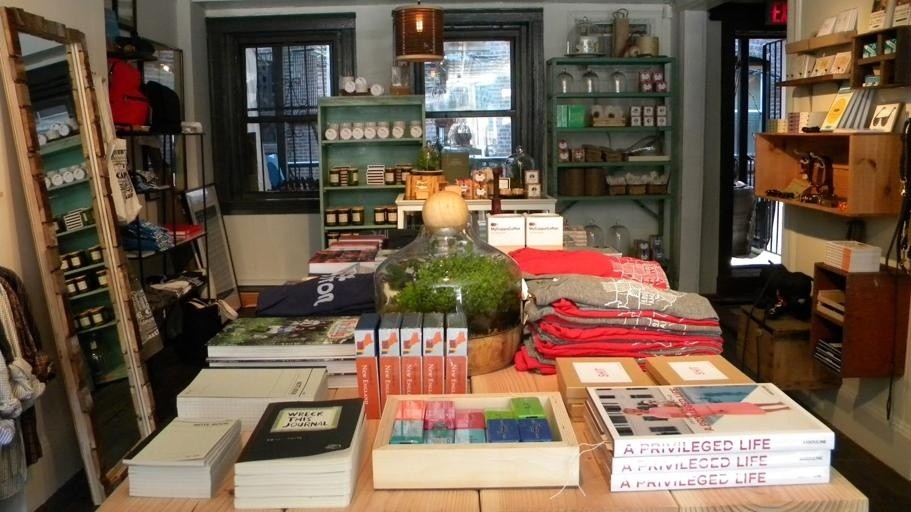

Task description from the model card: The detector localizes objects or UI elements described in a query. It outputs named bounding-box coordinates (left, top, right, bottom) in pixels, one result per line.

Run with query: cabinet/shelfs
left=116, top=133, right=208, bottom=369
left=546, top=57, right=676, bottom=282
left=809, top=262, right=910, bottom=377
left=16, top=47, right=129, bottom=387
left=317, top=95, right=425, bottom=252
left=753, top=1, right=910, bottom=219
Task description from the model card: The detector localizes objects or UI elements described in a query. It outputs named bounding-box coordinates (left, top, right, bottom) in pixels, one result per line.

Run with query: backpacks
left=106, top=55, right=149, bottom=128
left=145, top=80, right=183, bottom=136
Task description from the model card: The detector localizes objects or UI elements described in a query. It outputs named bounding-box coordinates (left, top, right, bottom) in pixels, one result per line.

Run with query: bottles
left=60, top=246, right=103, bottom=270
left=346, top=168, right=359, bottom=186
left=400, top=167, right=411, bottom=185
left=374, top=204, right=397, bottom=225
left=383, top=168, right=397, bottom=186
left=328, top=168, right=340, bottom=186
left=324, top=207, right=366, bottom=227
left=63, top=268, right=110, bottom=297
left=72, top=306, right=109, bottom=332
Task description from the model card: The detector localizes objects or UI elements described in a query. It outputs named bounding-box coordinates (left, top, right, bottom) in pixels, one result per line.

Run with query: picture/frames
left=183, top=184, right=242, bottom=326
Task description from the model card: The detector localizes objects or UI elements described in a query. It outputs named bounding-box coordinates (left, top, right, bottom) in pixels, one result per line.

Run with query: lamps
left=392, top=1, right=445, bottom=62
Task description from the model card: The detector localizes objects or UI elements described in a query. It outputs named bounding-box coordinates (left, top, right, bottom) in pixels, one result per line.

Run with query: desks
left=96, top=382, right=479, bottom=512
left=468, top=363, right=870, bottom=512
left=393, top=192, right=557, bottom=230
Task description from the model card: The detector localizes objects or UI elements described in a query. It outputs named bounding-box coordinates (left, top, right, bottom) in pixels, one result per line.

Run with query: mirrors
left=0, top=6, right=155, bottom=506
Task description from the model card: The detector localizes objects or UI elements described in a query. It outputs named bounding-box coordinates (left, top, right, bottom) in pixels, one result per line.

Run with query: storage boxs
left=736, top=305, right=826, bottom=395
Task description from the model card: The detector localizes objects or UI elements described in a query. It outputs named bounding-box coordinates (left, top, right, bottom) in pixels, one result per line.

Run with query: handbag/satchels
left=105, top=136, right=145, bottom=226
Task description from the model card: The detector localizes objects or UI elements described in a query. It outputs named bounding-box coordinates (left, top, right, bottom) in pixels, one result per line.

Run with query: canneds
left=401, top=166, right=412, bottom=183
left=352, top=208, right=364, bottom=224
left=387, top=207, right=397, bottom=224
left=328, top=234, right=338, bottom=245
left=71, top=304, right=107, bottom=331
left=330, top=168, right=341, bottom=186
left=338, top=208, right=348, bottom=224
left=348, top=167, right=360, bottom=184
left=57, top=245, right=112, bottom=293
left=384, top=168, right=395, bottom=184
left=326, top=209, right=335, bottom=225
left=373, top=208, right=386, bottom=225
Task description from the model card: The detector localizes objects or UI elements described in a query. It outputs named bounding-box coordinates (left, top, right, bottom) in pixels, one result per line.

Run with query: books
left=120, top=312, right=843, bottom=509
left=821, top=87, right=875, bottom=132
left=309, top=233, right=386, bottom=278
left=824, top=242, right=882, bottom=271
